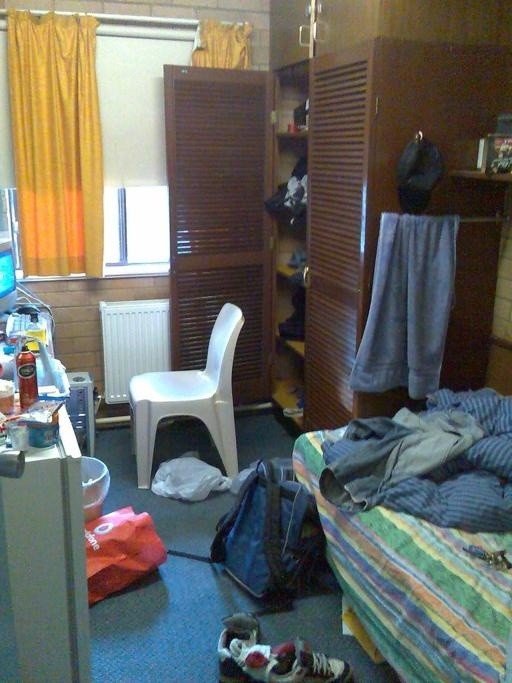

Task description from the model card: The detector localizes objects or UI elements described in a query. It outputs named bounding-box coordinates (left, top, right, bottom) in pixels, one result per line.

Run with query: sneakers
left=217, top=612, right=353, bottom=683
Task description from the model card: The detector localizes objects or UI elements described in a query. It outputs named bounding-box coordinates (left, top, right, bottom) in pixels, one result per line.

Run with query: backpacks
left=210, top=458, right=324, bottom=610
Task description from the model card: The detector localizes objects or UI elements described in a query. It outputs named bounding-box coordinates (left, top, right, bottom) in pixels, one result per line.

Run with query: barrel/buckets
left=80, top=455, right=109, bottom=523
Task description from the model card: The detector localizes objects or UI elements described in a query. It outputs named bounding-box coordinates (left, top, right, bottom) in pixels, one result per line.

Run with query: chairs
left=128, top=302, right=245, bottom=490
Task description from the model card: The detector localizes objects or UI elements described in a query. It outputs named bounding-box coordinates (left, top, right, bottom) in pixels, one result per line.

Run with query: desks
left=0, top=384, right=91, bottom=683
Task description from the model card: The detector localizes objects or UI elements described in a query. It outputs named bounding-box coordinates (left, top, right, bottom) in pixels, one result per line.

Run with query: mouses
left=15, top=305, right=40, bottom=313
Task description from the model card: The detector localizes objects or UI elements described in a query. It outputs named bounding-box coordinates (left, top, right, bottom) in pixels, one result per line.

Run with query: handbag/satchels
left=84, top=505, right=167, bottom=608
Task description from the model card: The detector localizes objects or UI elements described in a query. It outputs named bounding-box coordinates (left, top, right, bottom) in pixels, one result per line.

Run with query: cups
left=11, top=424, right=29, bottom=451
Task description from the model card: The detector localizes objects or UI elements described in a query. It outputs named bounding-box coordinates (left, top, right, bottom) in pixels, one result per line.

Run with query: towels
left=347, top=212, right=460, bottom=401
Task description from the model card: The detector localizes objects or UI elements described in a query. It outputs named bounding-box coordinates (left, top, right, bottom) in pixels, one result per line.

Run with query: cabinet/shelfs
left=163, top=2, right=512, bottom=439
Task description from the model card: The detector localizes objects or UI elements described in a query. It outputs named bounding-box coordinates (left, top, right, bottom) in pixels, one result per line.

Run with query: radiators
left=98, top=292, right=251, bottom=406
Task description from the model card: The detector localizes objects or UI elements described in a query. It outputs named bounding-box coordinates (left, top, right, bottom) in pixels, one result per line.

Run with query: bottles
left=0, top=313, right=46, bottom=414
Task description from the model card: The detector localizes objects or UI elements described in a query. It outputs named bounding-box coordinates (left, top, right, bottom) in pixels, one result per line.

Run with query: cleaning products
left=15, top=304, right=47, bottom=352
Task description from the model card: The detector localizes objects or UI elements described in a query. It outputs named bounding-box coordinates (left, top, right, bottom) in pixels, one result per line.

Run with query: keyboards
left=6, top=312, right=48, bottom=350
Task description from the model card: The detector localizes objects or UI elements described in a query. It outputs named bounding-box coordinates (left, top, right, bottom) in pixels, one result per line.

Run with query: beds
left=292, top=388, right=512, bottom=682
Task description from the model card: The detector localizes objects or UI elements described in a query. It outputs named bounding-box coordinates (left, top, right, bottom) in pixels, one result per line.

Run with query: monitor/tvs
left=0, top=238, right=18, bottom=315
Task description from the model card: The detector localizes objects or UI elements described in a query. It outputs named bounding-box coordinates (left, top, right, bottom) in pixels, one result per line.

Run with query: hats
left=398, top=134, right=443, bottom=214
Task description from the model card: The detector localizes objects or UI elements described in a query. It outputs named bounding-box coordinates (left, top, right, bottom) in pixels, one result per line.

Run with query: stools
left=66, top=367, right=95, bottom=457
